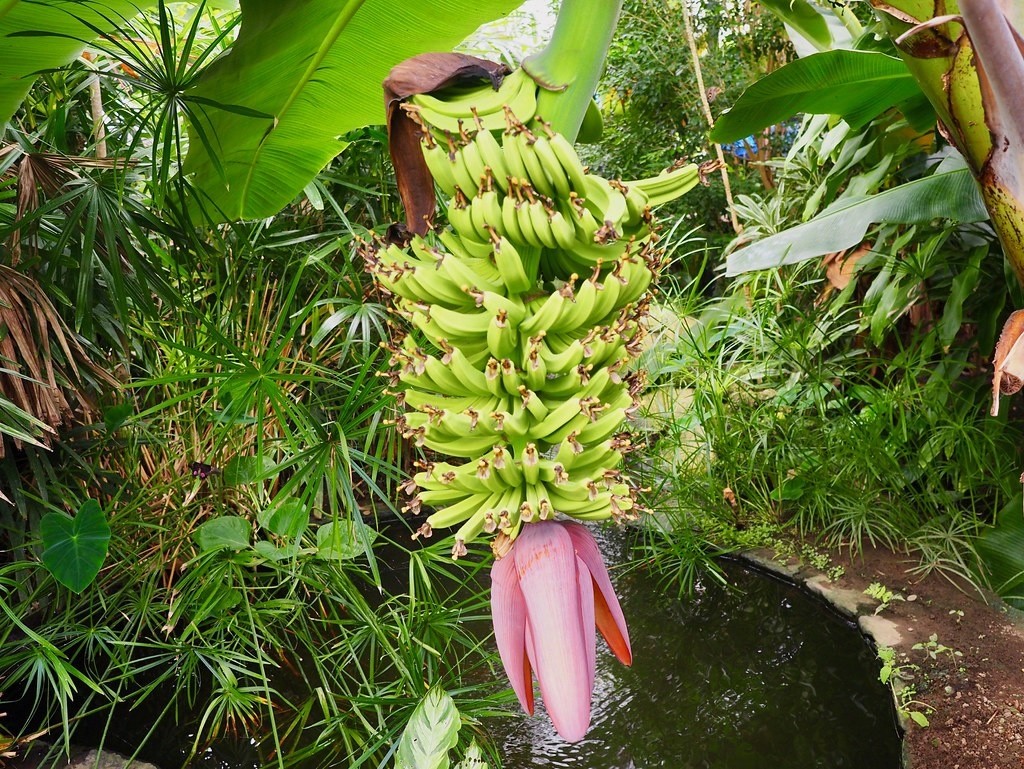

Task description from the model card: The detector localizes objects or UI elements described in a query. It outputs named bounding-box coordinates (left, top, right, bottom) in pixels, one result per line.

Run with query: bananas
left=355, top=65, right=722, bottom=564
